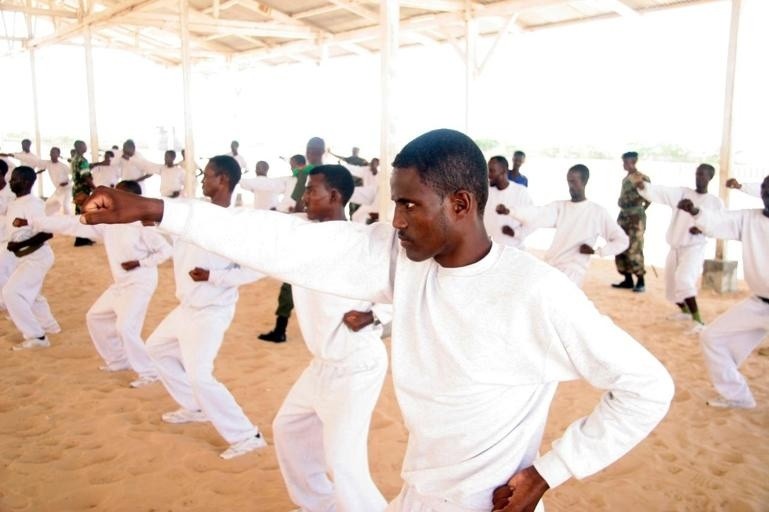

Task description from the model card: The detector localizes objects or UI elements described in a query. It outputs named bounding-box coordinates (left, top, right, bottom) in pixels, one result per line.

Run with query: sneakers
left=632, top=283, right=646, bottom=292
left=128, top=374, right=159, bottom=388
left=161, top=408, right=208, bottom=423
left=45, top=324, right=61, bottom=334
left=705, top=398, right=756, bottom=410
left=98, top=366, right=128, bottom=373
left=218, top=432, right=268, bottom=460
left=684, top=320, right=704, bottom=336
left=664, top=313, right=692, bottom=322
left=611, top=281, right=634, bottom=289
left=12, top=336, right=50, bottom=351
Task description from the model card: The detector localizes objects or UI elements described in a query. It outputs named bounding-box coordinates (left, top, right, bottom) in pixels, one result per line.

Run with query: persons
left=114, top=139, right=153, bottom=182
left=223, top=140, right=246, bottom=171
left=327, top=146, right=368, bottom=222
left=337, top=157, right=380, bottom=222
left=88, top=151, right=114, bottom=190
left=241, top=161, right=278, bottom=212
left=71, top=141, right=96, bottom=246
left=0, top=168, right=62, bottom=352
left=289, top=154, right=305, bottom=174
left=508, top=150, right=528, bottom=188
left=677, top=175, right=769, bottom=410
left=12, top=181, right=174, bottom=389
left=0, top=159, right=15, bottom=305
left=142, top=156, right=268, bottom=460
left=25, top=147, right=71, bottom=217
left=190, top=165, right=390, bottom=512
left=131, top=150, right=183, bottom=198
left=483, top=156, right=540, bottom=249
left=611, top=152, right=653, bottom=293
left=257, top=136, right=324, bottom=342
left=725, top=178, right=761, bottom=198
left=80, top=129, right=674, bottom=512
left=496, top=163, right=629, bottom=288
left=175, top=148, right=202, bottom=197
left=0, top=140, right=37, bottom=172
left=629, top=164, right=724, bottom=324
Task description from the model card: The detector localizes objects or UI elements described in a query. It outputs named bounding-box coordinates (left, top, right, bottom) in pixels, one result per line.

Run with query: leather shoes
left=258, top=331, right=286, bottom=344
